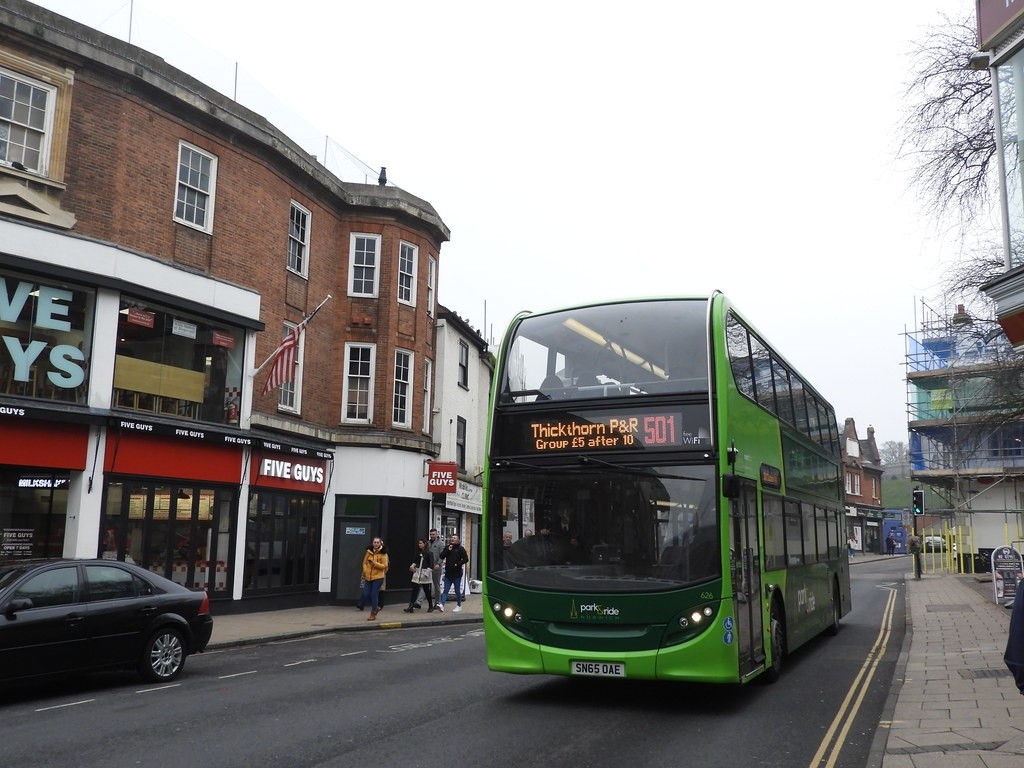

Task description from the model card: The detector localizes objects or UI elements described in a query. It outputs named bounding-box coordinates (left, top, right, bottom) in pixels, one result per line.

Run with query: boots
left=404, top=602, right=413, bottom=613
left=427, top=603, right=433, bottom=612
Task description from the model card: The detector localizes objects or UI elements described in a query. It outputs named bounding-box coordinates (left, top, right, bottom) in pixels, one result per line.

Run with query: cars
left=921, top=536, right=946, bottom=553
left=0, top=557, right=214, bottom=694
left=952, top=543, right=957, bottom=561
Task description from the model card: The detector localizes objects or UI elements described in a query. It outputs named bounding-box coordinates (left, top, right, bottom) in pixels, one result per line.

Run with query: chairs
left=535, top=362, right=701, bottom=401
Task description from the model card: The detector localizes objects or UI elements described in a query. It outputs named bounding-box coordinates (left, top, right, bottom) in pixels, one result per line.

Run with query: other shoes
left=413, top=603, right=421, bottom=609
left=368, top=611, right=376, bottom=621
left=375, top=606, right=381, bottom=615
left=433, top=602, right=440, bottom=609
left=378, top=603, right=384, bottom=609
left=356, top=603, right=365, bottom=611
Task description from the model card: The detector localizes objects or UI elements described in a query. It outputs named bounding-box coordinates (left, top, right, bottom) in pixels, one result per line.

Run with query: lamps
left=177, top=489, right=190, bottom=500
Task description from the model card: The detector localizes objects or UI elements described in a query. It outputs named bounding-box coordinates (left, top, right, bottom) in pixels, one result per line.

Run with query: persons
left=524, top=529, right=532, bottom=537
left=355, top=536, right=389, bottom=621
left=846, top=536, right=857, bottom=558
left=403, top=528, right=469, bottom=613
left=885, top=534, right=896, bottom=556
left=502, top=531, right=513, bottom=546
left=541, top=500, right=584, bottom=566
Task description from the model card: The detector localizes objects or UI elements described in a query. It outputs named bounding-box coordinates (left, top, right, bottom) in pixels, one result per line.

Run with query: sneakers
left=453, top=605, right=461, bottom=612
left=436, top=604, right=445, bottom=612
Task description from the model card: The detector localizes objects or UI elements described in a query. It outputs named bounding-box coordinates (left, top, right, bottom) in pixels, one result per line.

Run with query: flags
left=260, top=307, right=318, bottom=400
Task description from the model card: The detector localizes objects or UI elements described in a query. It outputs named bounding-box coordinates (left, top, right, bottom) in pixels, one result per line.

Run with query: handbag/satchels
left=893, top=540, right=897, bottom=546
left=848, top=539, right=851, bottom=549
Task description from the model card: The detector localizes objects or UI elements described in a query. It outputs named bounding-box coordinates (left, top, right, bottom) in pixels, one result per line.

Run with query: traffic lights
left=914, top=491, right=924, bottom=516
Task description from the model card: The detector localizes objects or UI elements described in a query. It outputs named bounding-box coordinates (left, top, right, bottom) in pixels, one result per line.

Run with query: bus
left=481, top=288, right=851, bottom=691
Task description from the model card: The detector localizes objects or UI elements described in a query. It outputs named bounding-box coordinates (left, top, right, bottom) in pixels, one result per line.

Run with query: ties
left=562, top=526, right=568, bottom=534
